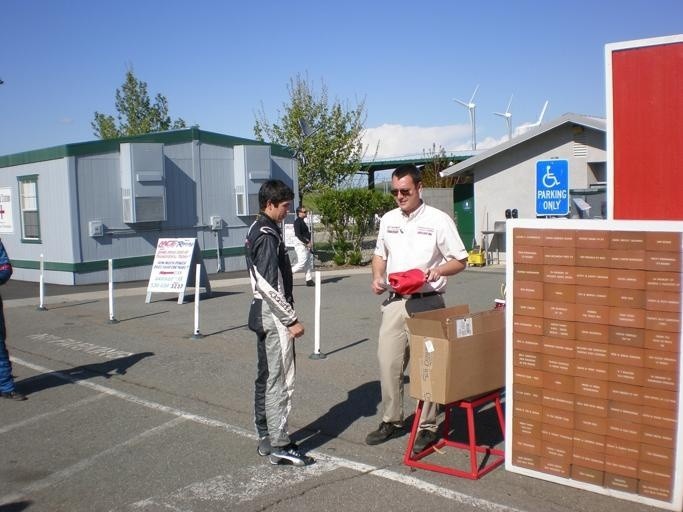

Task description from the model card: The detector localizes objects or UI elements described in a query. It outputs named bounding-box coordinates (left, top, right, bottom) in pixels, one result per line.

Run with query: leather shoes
left=389, top=290, right=442, bottom=298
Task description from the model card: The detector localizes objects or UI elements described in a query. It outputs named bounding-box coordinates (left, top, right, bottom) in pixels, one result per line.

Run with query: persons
left=0, top=239, right=23, bottom=400
left=366, top=163, right=467, bottom=453
left=244, top=181, right=315, bottom=465
left=289, top=206, right=315, bottom=287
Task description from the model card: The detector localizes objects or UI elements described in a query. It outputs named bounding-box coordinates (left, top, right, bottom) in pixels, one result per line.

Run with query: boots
left=2, top=390, right=25, bottom=401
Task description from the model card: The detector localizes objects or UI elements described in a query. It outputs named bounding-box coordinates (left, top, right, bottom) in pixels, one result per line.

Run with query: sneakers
left=306, top=280, right=315, bottom=286
left=366, top=422, right=439, bottom=454
left=258, top=436, right=314, bottom=466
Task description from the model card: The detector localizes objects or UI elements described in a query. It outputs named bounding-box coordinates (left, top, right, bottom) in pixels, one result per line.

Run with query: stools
left=403, top=388, right=504, bottom=480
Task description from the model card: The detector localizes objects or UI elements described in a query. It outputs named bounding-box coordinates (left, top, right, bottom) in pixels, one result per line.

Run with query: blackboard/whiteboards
left=147, top=237, right=196, bottom=293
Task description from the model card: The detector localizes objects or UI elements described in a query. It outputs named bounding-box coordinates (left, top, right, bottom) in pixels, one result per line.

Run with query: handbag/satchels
left=405, top=296, right=447, bottom=317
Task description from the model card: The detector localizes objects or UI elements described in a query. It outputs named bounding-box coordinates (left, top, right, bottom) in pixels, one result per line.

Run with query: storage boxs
left=403, top=302, right=505, bottom=405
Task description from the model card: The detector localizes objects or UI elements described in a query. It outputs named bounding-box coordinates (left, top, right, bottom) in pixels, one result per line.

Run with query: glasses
left=300, top=211, right=306, bottom=213
